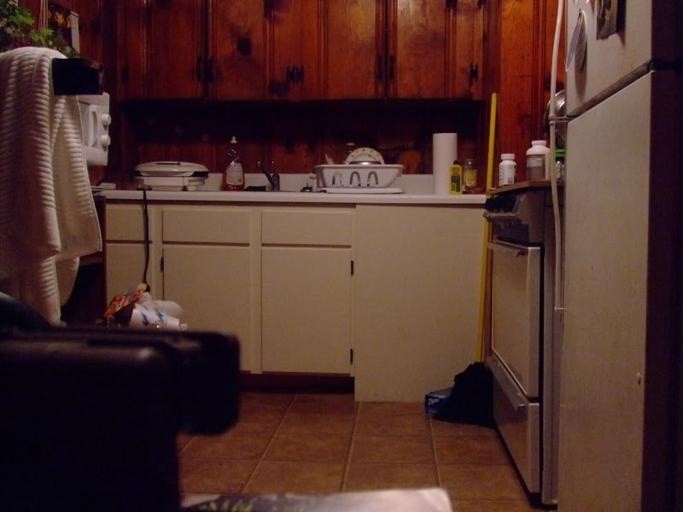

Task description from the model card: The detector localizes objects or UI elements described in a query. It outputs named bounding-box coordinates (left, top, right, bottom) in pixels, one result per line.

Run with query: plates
left=346, top=147, right=383, bottom=166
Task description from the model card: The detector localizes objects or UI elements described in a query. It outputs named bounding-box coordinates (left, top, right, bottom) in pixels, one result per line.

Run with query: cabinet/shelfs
left=61, top=1, right=205, bottom=101
left=204, top=1, right=322, bottom=100
left=451, top=1, right=499, bottom=101
left=322, top=1, right=451, bottom=100
left=160, top=205, right=351, bottom=374
left=103, top=202, right=160, bottom=311
left=478, top=1, right=565, bottom=193
left=351, top=203, right=491, bottom=409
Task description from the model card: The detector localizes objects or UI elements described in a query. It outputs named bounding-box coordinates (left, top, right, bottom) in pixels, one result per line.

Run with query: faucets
left=257, top=156, right=281, bottom=192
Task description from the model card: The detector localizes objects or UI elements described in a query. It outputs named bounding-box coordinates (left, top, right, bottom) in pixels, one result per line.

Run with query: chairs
left=0, top=290, right=246, bottom=512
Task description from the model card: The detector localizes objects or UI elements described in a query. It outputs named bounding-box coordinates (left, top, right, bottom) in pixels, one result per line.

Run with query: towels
left=0, top=48, right=103, bottom=325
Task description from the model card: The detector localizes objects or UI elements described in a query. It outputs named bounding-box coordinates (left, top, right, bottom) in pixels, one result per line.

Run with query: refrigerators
left=551, top=0, right=681, bottom=509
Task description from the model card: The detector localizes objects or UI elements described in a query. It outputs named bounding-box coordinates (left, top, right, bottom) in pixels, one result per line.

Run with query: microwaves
left=74, top=90, right=113, bottom=166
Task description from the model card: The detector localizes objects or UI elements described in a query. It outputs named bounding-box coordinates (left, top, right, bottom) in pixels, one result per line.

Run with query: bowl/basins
left=542, top=91, right=565, bottom=123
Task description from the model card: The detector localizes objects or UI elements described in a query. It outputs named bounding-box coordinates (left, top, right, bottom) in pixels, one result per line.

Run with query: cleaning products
left=449, top=158, right=479, bottom=195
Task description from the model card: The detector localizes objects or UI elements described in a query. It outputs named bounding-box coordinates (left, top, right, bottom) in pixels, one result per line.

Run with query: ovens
left=484, top=238, right=551, bottom=504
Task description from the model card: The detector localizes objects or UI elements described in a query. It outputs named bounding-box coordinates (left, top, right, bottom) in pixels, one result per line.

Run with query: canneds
left=554, top=150, right=565, bottom=179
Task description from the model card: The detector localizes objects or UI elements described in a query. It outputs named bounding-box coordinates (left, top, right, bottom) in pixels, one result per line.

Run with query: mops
left=432, top=92, right=500, bottom=426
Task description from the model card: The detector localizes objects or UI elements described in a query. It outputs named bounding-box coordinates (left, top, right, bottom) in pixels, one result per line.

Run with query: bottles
left=343, top=142, right=354, bottom=161
left=462, top=156, right=475, bottom=190
left=449, top=159, right=461, bottom=194
left=526, top=141, right=550, bottom=180
left=498, top=152, right=519, bottom=187
left=555, top=149, right=565, bottom=185
